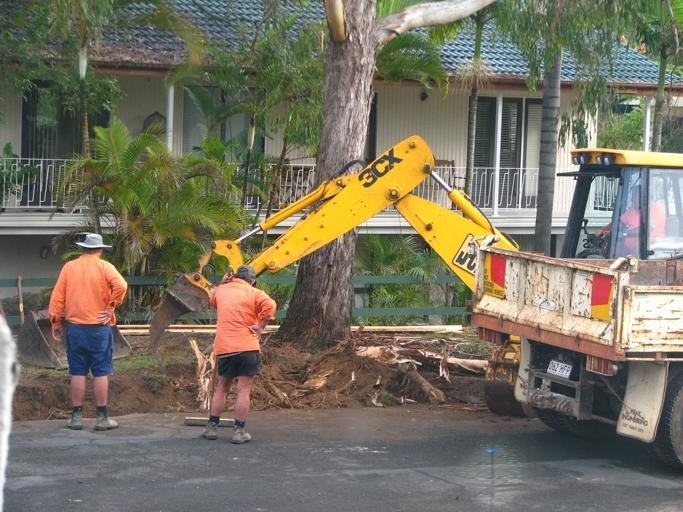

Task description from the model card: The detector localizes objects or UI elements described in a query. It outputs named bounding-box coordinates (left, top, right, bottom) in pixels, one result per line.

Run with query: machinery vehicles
left=139, top=131, right=683, bottom=420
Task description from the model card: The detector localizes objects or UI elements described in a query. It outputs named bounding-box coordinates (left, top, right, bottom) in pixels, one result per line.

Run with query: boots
left=232, top=425, right=252, bottom=443
left=67, top=412, right=85, bottom=429
left=203, top=421, right=220, bottom=440
left=95, top=409, right=119, bottom=430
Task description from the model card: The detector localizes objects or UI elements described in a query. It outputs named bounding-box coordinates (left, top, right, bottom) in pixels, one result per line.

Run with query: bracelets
left=622, top=229, right=627, bottom=239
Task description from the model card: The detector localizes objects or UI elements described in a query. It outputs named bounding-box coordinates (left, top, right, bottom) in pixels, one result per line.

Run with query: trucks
left=469, top=241, right=683, bottom=468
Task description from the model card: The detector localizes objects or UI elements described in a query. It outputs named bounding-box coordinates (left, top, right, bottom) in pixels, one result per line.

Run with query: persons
left=46, top=232, right=128, bottom=431
left=593, top=181, right=666, bottom=262
left=201, top=264, right=276, bottom=444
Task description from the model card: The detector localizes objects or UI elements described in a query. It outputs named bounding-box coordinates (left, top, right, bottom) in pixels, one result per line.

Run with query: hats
left=237, top=265, right=257, bottom=286
left=76, top=233, right=112, bottom=248
